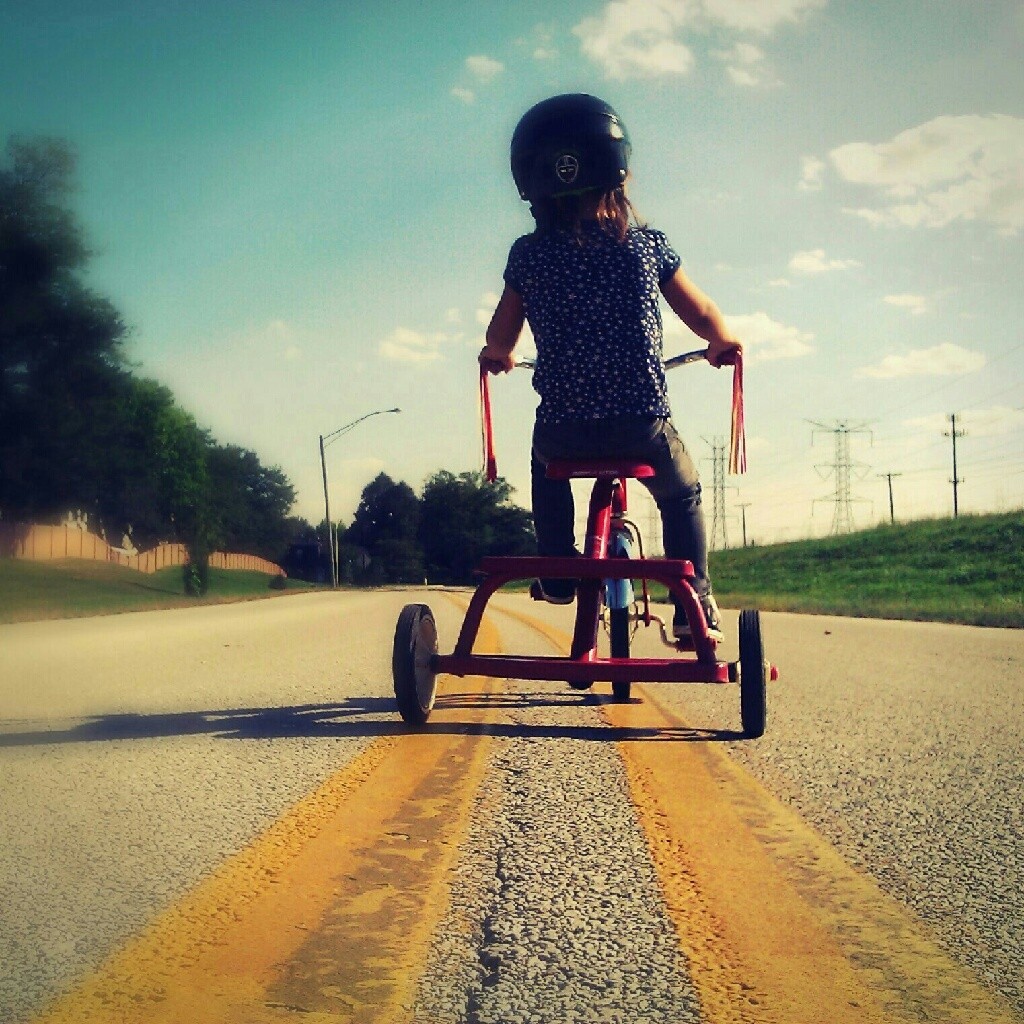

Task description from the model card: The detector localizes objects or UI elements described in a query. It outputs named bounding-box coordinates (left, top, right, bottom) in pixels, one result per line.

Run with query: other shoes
left=673, top=606, right=720, bottom=636
left=537, top=577, right=574, bottom=605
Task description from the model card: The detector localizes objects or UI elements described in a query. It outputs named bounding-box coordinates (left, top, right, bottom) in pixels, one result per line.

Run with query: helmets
left=510, top=94, right=628, bottom=199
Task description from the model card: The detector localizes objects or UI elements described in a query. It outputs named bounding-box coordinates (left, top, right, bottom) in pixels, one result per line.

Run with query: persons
left=479, top=94, right=742, bottom=643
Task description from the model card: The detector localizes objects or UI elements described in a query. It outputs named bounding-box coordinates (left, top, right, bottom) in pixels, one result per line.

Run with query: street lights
left=734, top=503, right=751, bottom=546
left=320, top=408, right=402, bottom=586
left=878, top=474, right=900, bottom=524
left=942, top=411, right=967, bottom=519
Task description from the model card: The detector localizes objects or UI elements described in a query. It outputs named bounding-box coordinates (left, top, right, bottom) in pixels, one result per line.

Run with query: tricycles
left=393, top=342, right=776, bottom=738
